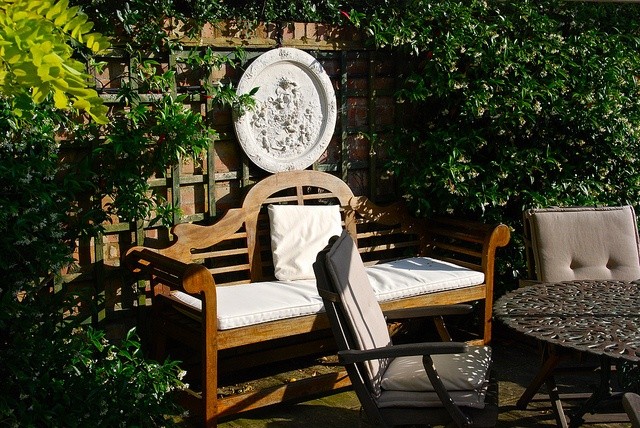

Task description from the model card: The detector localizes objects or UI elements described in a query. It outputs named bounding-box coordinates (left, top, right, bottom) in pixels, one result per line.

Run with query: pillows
left=267, top=203, right=342, bottom=281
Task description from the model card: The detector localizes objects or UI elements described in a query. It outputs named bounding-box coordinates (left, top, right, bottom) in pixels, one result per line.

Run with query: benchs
left=124, top=171, right=512, bottom=428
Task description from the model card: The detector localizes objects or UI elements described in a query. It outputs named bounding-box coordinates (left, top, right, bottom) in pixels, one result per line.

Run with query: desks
left=492, top=279, right=639, bottom=427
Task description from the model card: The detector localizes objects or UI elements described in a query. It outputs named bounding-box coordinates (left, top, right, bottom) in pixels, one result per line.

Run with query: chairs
left=312, top=228, right=499, bottom=427
left=516, top=205, right=640, bottom=428
